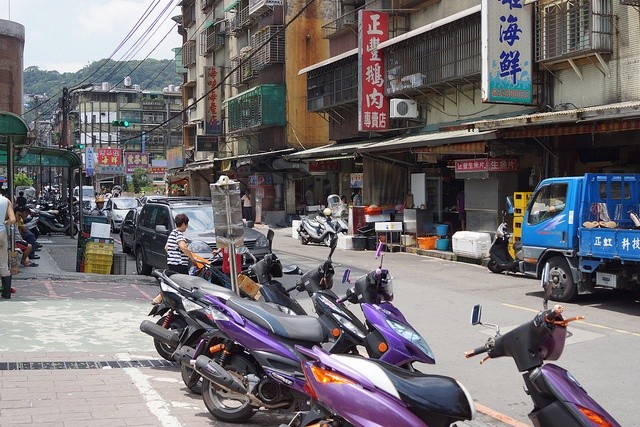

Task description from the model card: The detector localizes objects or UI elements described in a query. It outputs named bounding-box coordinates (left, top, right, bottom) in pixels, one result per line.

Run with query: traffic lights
left=73, top=144, right=84, bottom=148
left=112, top=120, right=129, bottom=126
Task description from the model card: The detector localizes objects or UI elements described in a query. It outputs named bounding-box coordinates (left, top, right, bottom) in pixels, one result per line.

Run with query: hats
left=95, top=196, right=105, bottom=202
left=0, top=175, right=8, bottom=181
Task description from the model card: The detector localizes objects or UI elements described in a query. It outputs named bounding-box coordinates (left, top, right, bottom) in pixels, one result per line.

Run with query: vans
left=73, top=185, right=96, bottom=209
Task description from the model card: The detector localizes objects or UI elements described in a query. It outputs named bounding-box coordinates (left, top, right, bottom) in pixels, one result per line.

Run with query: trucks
left=521, top=172, right=639, bottom=301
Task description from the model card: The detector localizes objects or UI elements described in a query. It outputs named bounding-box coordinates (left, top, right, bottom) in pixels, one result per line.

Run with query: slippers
left=152, top=302, right=162, bottom=305
left=29, top=262, right=38, bottom=266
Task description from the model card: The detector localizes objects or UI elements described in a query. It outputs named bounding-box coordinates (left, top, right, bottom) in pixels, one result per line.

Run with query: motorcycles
left=297, top=208, right=337, bottom=247
left=139, top=228, right=307, bottom=361
left=487, top=209, right=523, bottom=274
left=22, top=190, right=78, bottom=235
left=289, top=261, right=620, bottom=426
left=189, top=241, right=436, bottom=423
left=327, top=193, right=348, bottom=235
left=173, top=232, right=370, bottom=394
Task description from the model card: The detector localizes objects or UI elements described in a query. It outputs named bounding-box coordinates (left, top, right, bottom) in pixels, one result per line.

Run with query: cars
left=119, top=207, right=141, bottom=254
left=106, top=197, right=142, bottom=233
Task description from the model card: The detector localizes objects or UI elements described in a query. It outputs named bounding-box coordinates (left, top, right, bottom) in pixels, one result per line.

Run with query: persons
left=341, top=195, right=346, bottom=203
left=0, top=181, right=16, bottom=298
left=457, top=187, right=466, bottom=231
left=10, top=207, right=32, bottom=266
left=212, top=247, right=242, bottom=278
left=165, top=213, right=197, bottom=274
left=305, top=184, right=315, bottom=206
left=39, top=185, right=59, bottom=201
left=18, top=207, right=42, bottom=259
left=0, top=181, right=6, bottom=195
left=168, top=186, right=172, bottom=196
left=352, top=188, right=361, bottom=206
left=241, top=190, right=253, bottom=221
left=17, top=191, right=27, bottom=207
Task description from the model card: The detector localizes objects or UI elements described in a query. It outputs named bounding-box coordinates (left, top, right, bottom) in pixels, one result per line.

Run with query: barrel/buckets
left=432, top=224, right=448, bottom=237
left=436, top=239, right=449, bottom=250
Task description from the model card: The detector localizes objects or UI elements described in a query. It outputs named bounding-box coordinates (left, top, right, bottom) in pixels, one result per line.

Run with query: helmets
left=323, top=207, right=331, bottom=215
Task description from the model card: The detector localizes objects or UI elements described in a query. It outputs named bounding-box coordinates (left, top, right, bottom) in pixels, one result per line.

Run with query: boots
left=0, top=275, right=12, bottom=298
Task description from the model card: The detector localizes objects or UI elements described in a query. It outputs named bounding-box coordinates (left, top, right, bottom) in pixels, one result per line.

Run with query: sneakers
left=30, top=256, right=40, bottom=259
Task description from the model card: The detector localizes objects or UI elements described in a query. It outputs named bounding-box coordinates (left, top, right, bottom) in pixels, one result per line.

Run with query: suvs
left=132, top=196, right=271, bottom=275
